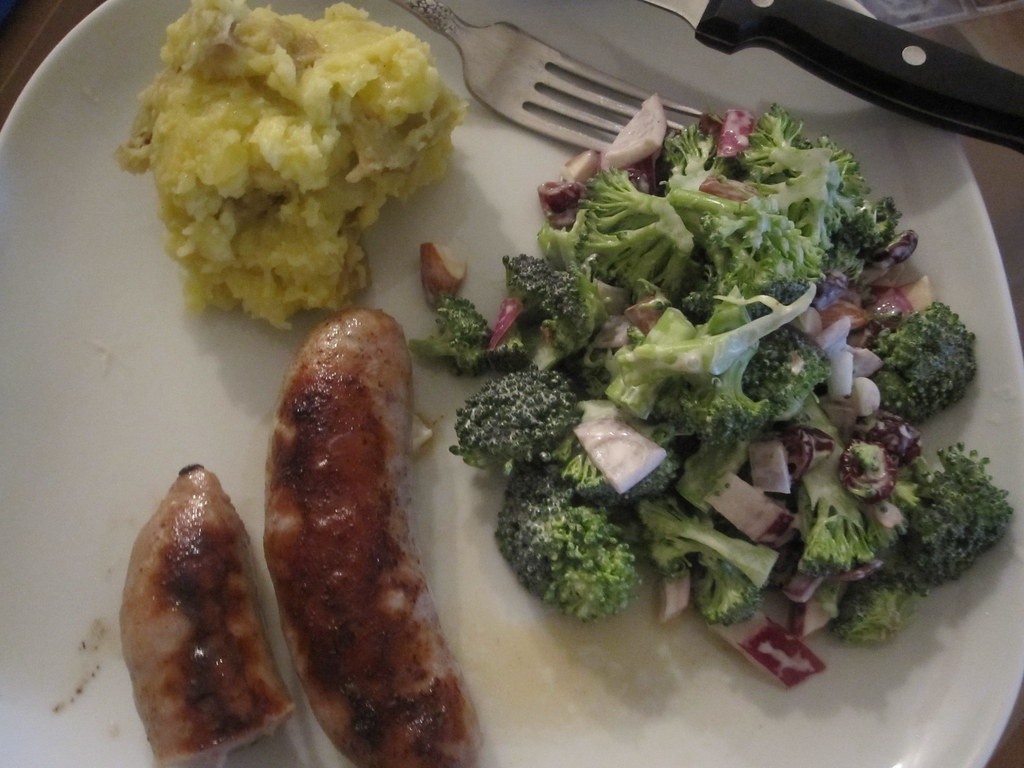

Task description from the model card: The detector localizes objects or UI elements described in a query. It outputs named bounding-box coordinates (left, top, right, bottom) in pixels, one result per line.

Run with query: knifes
left=643, top=0, right=1024, bottom=153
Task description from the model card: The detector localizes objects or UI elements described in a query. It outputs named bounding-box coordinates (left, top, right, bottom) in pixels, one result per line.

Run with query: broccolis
left=405, top=104, right=1014, bottom=646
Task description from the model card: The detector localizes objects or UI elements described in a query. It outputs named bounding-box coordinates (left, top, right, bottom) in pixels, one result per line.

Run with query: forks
left=395, top=0, right=704, bottom=169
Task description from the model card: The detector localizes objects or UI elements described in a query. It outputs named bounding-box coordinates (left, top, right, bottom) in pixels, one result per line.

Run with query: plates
left=0, top=0, right=1024, bottom=768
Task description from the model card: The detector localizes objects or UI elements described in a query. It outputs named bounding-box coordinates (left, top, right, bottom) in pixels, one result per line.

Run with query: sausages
left=119, top=464, right=296, bottom=768
left=262, top=307, right=486, bottom=768
